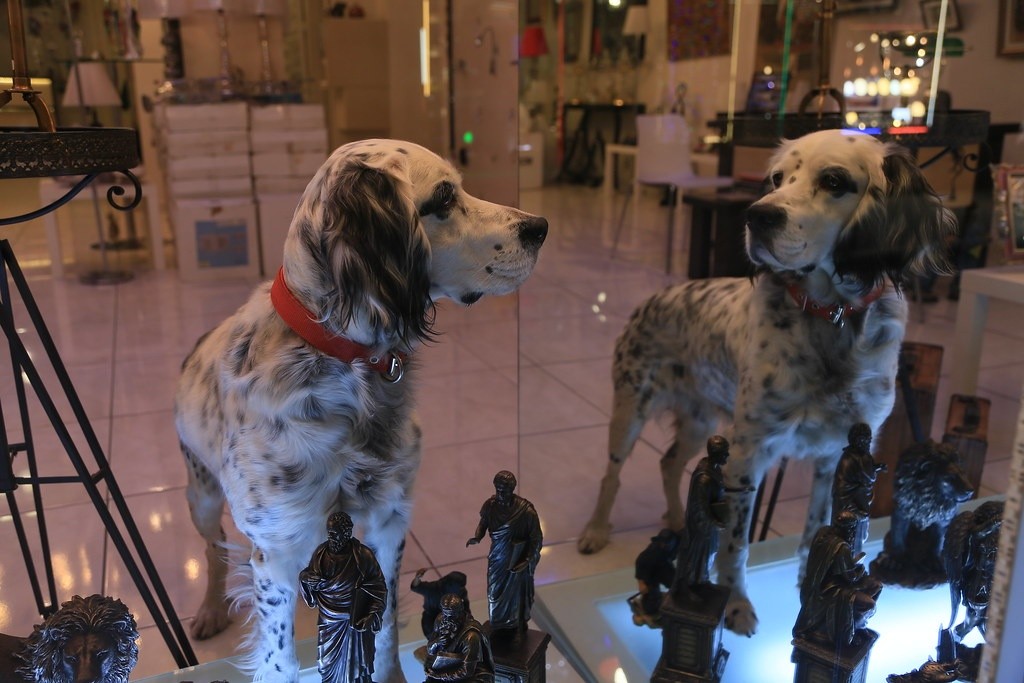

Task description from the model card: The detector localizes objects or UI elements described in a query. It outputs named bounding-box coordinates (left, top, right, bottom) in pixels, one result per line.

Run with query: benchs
left=683, top=189, right=767, bottom=280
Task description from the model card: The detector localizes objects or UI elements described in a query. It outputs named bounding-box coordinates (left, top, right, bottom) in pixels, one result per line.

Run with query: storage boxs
left=151, top=102, right=330, bottom=285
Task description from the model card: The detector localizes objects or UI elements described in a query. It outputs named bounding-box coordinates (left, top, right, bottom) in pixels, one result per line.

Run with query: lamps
left=63, top=62, right=124, bottom=251
left=200, top=0, right=245, bottom=99
left=238, top=0, right=284, bottom=92
left=520, top=27, right=548, bottom=79
left=474, top=28, right=499, bottom=56
left=140, top=0, right=194, bottom=85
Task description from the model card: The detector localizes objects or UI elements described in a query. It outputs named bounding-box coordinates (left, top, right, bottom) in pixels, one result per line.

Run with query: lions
left=880, top=439, right=975, bottom=571
left=0, top=594, right=140, bottom=683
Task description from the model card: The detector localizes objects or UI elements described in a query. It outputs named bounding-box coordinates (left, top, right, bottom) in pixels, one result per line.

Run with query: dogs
left=176, top=138, right=550, bottom=683
left=575, top=129, right=957, bottom=555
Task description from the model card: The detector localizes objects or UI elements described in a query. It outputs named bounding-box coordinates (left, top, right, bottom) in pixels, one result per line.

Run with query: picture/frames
left=993, top=163, right=1024, bottom=260
left=996, top=0, right=1024, bottom=58
left=920, top=0, right=963, bottom=34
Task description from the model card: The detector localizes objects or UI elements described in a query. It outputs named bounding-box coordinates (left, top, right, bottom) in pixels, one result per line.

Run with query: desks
left=930, top=264, right=1024, bottom=444
left=561, top=101, right=645, bottom=187
left=531, top=491, right=1007, bottom=683
left=136, top=598, right=491, bottom=683
left=704, top=111, right=992, bottom=271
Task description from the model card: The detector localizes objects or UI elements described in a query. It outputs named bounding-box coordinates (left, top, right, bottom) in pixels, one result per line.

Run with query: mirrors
left=520, top=0, right=1024, bottom=683
